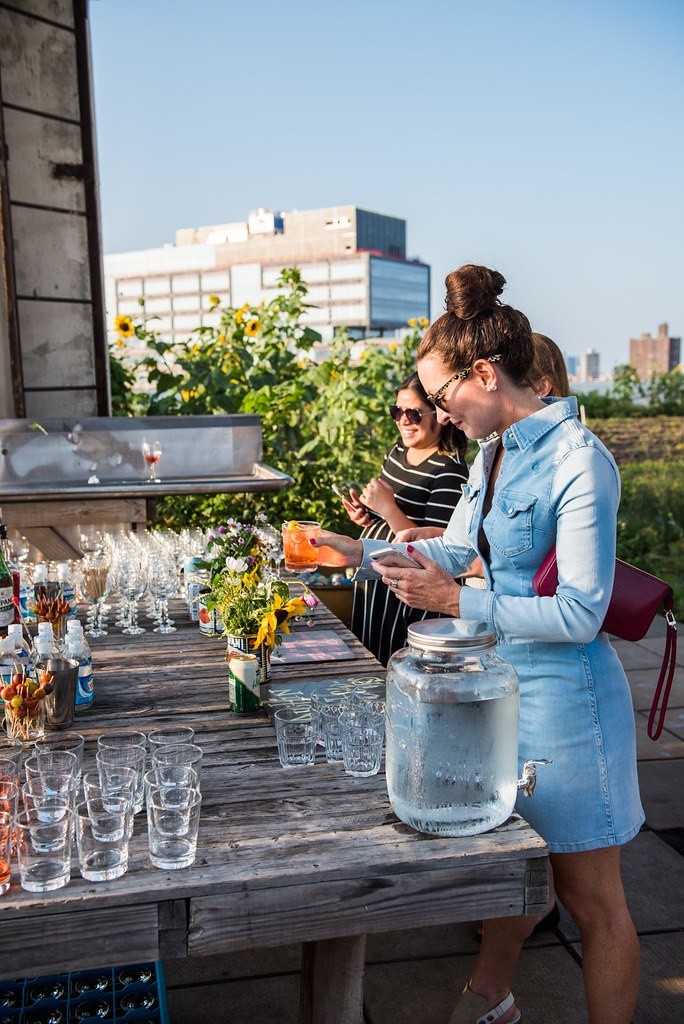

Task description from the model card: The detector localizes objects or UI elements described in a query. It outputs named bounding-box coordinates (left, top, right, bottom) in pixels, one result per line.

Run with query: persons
left=283, top=264, right=646, bottom=1024
left=342, top=370, right=470, bottom=669
left=387, top=332, right=569, bottom=940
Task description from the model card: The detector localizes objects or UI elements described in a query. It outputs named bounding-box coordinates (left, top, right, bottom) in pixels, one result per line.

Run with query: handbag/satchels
left=532, top=536, right=679, bottom=743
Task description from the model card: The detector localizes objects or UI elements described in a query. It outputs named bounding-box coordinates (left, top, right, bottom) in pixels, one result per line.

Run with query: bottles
left=0, top=508, right=77, bottom=648
left=0, top=619, right=94, bottom=723
left=384, top=618, right=553, bottom=838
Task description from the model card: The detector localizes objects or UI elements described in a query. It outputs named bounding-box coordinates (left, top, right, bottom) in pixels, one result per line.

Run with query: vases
left=227, top=634, right=274, bottom=684
left=195, top=595, right=227, bottom=638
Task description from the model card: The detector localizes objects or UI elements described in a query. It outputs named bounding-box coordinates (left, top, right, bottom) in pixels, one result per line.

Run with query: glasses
left=389, top=404, right=437, bottom=427
left=425, top=364, right=473, bottom=414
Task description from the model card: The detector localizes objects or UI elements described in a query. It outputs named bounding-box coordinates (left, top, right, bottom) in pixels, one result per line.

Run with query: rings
left=390, top=580, right=398, bottom=588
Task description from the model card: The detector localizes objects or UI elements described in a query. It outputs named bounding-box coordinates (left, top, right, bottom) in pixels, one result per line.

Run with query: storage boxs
left=0, top=962, right=169, bottom=1024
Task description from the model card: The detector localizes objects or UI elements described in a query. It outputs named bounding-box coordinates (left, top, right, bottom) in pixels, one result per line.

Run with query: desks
left=0, top=580, right=551, bottom=1024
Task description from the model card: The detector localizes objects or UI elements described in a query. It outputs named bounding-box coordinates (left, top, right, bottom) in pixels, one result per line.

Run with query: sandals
left=476, top=989, right=522, bottom=1024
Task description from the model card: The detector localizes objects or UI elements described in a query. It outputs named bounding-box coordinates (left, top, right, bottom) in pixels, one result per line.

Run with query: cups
left=274, top=683, right=386, bottom=778
left=281, top=521, right=321, bottom=574
left=34, top=658, right=80, bottom=730
left=0, top=725, right=203, bottom=897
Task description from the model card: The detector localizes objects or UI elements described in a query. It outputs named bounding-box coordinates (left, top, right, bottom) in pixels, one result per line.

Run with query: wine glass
left=80, top=525, right=285, bottom=638
left=141, top=440, right=162, bottom=484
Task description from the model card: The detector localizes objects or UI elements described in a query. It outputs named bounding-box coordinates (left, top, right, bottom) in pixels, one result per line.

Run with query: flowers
left=187, top=507, right=322, bottom=666
